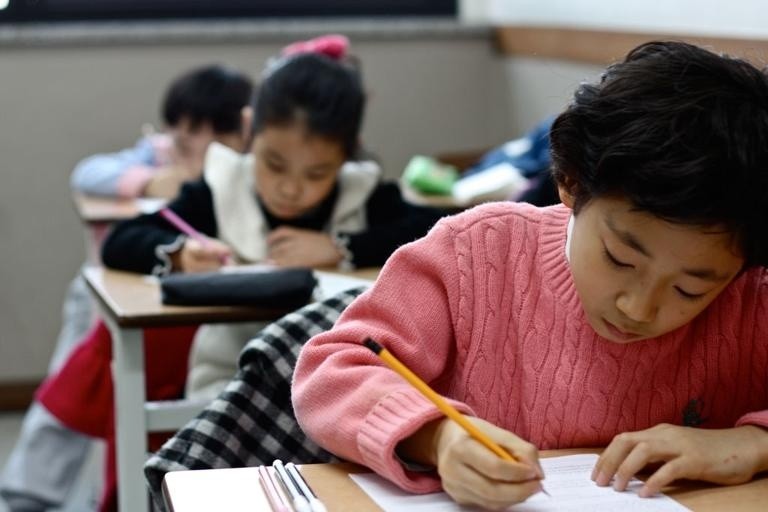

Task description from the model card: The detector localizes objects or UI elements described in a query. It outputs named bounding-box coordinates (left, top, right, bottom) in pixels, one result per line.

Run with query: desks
left=71, top=178, right=506, bottom=264
left=159, top=448, right=768, bottom=512
left=81, top=261, right=382, bottom=512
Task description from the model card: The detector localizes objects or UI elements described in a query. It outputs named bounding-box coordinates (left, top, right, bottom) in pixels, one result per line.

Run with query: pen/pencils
left=159, top=207, right=239, bottom=266
left=362, top=337, right=550, bottom=495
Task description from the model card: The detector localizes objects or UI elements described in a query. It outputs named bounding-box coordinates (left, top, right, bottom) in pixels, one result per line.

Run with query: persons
left=287, top=38, right=767, bottom=512
left=94, top=29, right=418, bottom=402
left=0, top=56, right=262, bottom=512
